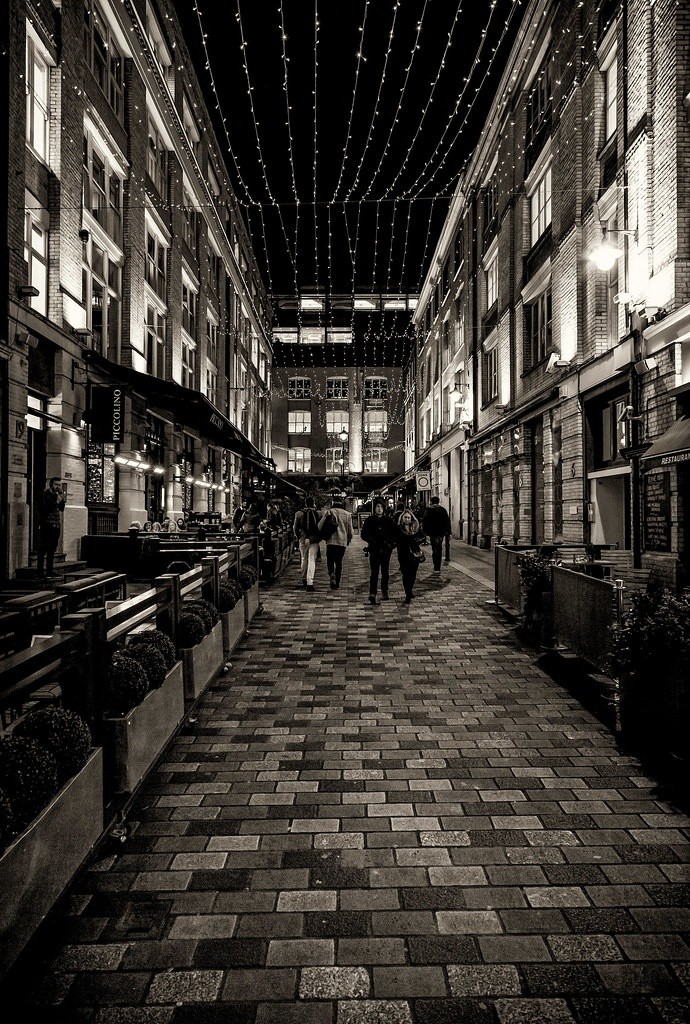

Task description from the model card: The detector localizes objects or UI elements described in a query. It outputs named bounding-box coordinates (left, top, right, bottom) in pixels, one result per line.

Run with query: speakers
left=634, top=357, right=656, bottom=375
left=19, top=332, right=39, bottom=348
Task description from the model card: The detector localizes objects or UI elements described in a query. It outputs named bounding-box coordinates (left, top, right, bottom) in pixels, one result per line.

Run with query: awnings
left=246, top=456, right=307, bottom=500
left=81, top=353, right=278, bottom=468
left=638, top=412, right=690, bottom=473
left=380, top=476, right=415, bottom=495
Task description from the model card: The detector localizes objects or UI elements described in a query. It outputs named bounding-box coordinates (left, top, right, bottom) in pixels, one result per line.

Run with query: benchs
left=0, top=571, right=128, bottom=659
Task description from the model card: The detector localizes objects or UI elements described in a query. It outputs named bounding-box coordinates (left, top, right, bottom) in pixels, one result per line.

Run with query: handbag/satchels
left=412, top=552, right=426, bottom=564
left=319, top=510, right=338, bottom=541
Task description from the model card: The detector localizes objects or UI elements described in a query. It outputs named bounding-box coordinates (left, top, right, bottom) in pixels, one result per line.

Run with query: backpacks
left=300, top=508, right=318, bottom=534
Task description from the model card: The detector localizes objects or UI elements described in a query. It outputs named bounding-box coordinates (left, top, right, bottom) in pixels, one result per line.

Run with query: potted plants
left=217, top=578, right=246, bottom=653
left=59, top=631, right=186, bottom=793
left=239, top=562, right=260, bottom=622
left=158, top=598, right=224, bottom=701
left=0, top=706, right=104, bottom=967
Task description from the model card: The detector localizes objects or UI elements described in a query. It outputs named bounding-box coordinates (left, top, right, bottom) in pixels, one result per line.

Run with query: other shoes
left=303, top=579, right=307, bottom=584
left=37, top=574, right=46, bottom=581
left=405, top=592, right=414, bottom=603
left=330, top=580, right=340, bottom=589
left=307, top=586, right=315, bottom=591
left=46, top=571, right=63, bottom=579
left=382, top=590, right=388, bottom=600
left=369, top=594, right=376, bottom=605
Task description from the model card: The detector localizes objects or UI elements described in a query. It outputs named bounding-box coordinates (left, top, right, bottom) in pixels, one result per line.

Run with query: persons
left=361, top=498, right=397, bottom=606
left=231, top=498, right=282, bottom=535
left=34, top=477, right=66, bottom=580
left=317, top=500, right=353, bottom=590
left=395, top=509, right=426, bottom=604
left=292, top=496, right=323, bottom=592
left=421, top=496, right=451, bottom=574
left=384, top=499, right=430, bottom=546
left=128, top=516, right=188, bottom=541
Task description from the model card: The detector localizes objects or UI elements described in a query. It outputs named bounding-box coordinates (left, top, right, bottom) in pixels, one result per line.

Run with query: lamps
left=469, top=453, right=522, bottom=477
left=448, top=383, right=469, bottom=402
left=635, top=357, right=657, bottom=374
left=173, top=473, right=230, bottom=493
left=74, top=326, right=92, bottom=337
left=83, top=448, right=164, bottom=480
left=22, top=284, right=38, bottom=297
left=588, top=225, right=636, bottom=273
left=19, top=332, right=39, bottom=348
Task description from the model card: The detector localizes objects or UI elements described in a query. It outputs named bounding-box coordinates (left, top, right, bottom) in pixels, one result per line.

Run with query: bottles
left=190, top=518, right=219, bottom=525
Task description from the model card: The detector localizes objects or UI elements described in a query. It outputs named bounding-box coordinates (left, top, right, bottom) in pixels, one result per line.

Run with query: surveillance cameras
left=613, top=292, right=641, bottom=305
left=545, top=353, right=560, bottom=374
